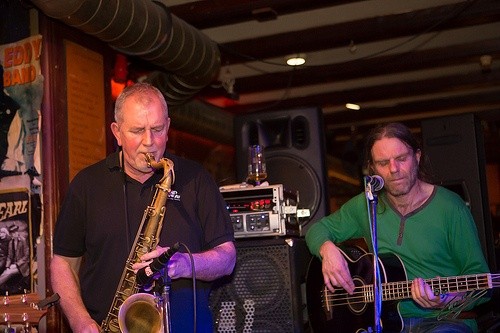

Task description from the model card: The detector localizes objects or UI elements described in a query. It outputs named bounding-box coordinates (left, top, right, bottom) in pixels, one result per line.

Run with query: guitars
left=305, top=243, right=500, bottom=333
left=0, top=289, right=61, bottom=333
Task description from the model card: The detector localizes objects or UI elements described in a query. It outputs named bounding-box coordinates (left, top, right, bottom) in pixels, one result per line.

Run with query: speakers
left=232, top=104, right=328, bottom=239
left=419, top=113, right=496, bottom=275
left=205, top=236, right=354, bottom=332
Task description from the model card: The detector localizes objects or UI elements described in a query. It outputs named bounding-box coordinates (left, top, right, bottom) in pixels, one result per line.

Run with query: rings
left=424, top=301, right=427, bottom=305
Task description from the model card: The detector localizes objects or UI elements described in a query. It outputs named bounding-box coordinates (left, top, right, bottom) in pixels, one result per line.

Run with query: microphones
left=365, top=174, right=384, bottom=192
left=135, top=245, right=178, bottom=285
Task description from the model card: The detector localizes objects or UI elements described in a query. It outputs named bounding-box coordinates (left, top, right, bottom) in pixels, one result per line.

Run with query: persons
left=49, top=82, right=236, bottom=333
left=306, top=127, right=491, bottom=333
left=0, top=224, right=29, bottom=285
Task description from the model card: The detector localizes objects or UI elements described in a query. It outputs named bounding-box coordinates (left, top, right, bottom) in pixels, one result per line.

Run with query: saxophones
left=100, top=152, right=175, bottom=333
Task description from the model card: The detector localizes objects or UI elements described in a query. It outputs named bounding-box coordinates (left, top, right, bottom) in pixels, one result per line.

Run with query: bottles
left=247, top=145, right=266, bottom=184
